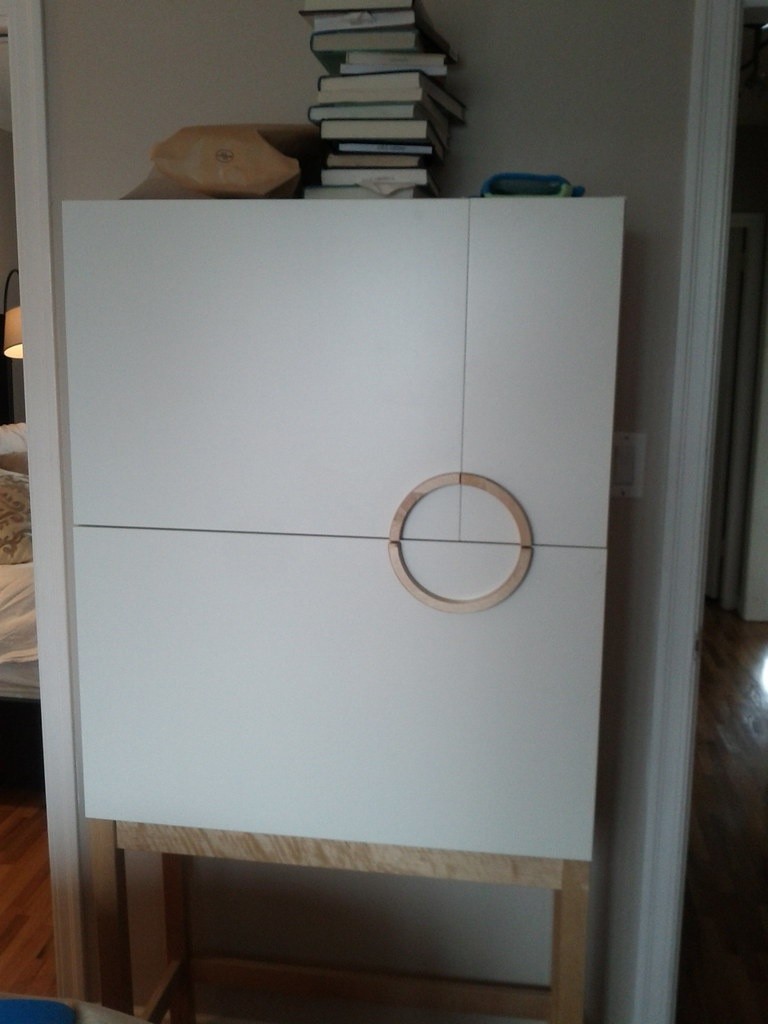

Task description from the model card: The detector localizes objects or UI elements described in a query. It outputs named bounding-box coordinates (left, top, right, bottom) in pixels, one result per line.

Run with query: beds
left=0, top=420, right=46, bottom=796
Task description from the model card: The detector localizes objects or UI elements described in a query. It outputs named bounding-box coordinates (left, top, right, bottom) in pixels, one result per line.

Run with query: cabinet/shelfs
left=51, top=193, right=628, bottom=1024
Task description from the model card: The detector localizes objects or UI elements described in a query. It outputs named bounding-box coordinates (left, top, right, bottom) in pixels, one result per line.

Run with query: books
left=298, top=0, right=468, bottom=198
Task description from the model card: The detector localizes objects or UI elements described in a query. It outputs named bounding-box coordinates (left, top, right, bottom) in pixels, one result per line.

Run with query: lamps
left=1, top=268, right=24, bottom=387
